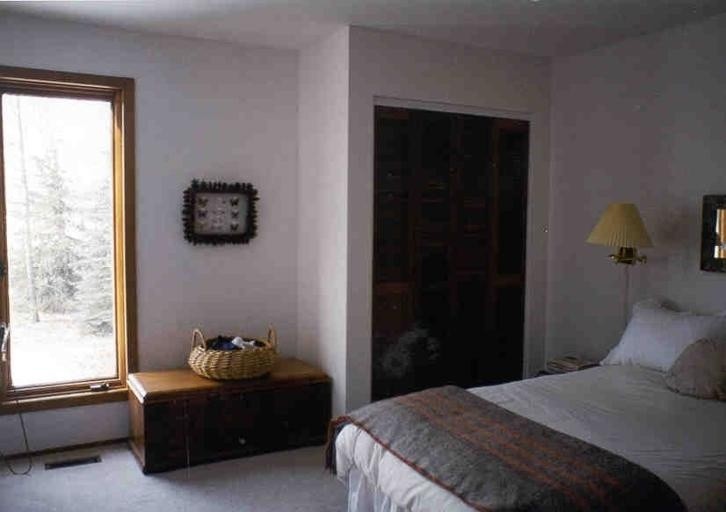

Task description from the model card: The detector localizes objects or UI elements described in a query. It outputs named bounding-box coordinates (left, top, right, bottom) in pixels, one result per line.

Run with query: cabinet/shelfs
left=125, top=357, right=333, bottom=474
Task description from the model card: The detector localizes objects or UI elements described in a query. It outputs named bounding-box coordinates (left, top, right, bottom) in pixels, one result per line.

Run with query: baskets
left=186, top=324, right=281, bottom=381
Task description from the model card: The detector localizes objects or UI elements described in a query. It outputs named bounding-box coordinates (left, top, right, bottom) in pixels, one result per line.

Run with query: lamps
left=583, top=202, right=656, bottom=353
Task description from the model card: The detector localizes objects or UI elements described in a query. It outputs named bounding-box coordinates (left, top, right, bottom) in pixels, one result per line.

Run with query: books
left=547, top=359, right=597, bottom=372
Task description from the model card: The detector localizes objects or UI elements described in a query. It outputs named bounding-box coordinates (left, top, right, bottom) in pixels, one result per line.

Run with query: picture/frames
left=180, top=177, right=260, bottom=245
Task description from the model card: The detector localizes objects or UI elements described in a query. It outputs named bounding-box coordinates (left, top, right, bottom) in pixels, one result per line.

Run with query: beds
left=325, top=362, right=726, bottom=510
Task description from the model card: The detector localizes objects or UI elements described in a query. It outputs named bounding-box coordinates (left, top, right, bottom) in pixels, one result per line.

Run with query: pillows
left=598, top=299, right=725, bottom=400
